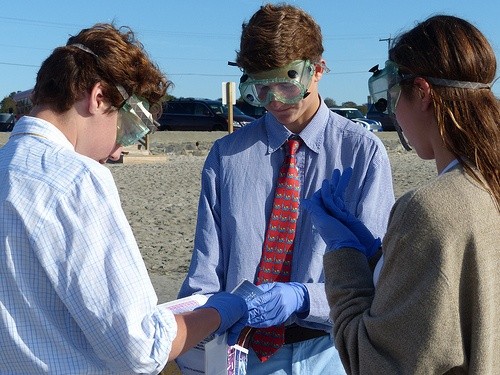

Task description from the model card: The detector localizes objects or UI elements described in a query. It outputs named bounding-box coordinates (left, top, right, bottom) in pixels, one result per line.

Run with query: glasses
left=118, top=95, right=160, bottom=147
left=367, top=59, right=403, bottom=114
left=239, top=59, right=317, bottom=108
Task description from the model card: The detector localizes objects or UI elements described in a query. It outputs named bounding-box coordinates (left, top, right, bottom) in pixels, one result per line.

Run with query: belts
left=285, top=323, right=330, bottom=344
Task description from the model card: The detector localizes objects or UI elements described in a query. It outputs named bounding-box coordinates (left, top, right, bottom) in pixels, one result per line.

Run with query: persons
left=177, top=3, right=396, bottom=375
left=0, top=23, right=249, bottom=374
left=300, top=15, right=500, bottom=375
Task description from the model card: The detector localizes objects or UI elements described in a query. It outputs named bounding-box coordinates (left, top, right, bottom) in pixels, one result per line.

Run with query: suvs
left=154, top=97, right=257, bottom=132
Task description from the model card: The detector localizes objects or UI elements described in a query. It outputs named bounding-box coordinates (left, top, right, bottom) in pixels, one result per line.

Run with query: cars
left=329, top=104, right=398, bottom=133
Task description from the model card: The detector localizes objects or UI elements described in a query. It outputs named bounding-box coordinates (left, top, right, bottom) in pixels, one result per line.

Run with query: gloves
left=301, top=167, right=368, bottom=259
left=321, top=168, right=382, bottom=256
left=194, top=292, right=248, bottom=346
left=246, top=281, right=309, bottom=328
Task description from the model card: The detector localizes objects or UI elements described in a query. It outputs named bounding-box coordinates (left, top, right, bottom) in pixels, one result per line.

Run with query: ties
left=251, top=139, right=301, bottom=363
